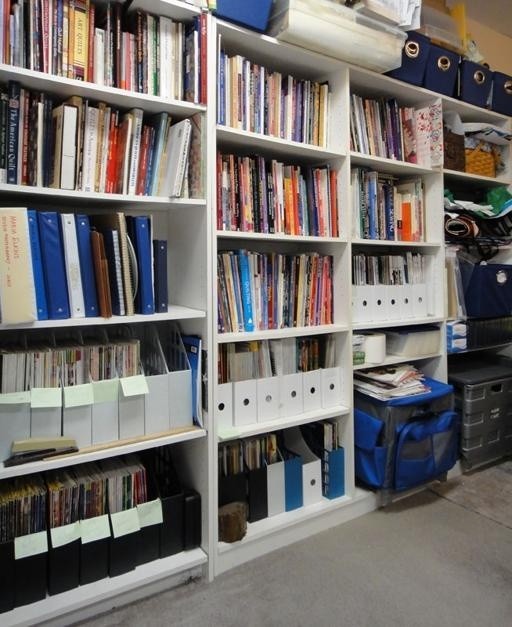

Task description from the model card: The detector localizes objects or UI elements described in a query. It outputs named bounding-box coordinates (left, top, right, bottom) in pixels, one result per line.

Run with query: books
left=218, top=434, right=277, bottom=478
left=2, top=208, right=168, bottom=324
left=216, top=149, right=339, bottom=238
left=218, top=333, right=336, bottom=385
left=351, top=165, right=426, bottom=243
left=2, top=454, right=146, bottom=542
left=299, top=419, right=340, bottom=496
left=353, top=363, right=431, bottom=401
left=2, top=337, right=140, bottom=394
left=2, top=80, right=206, bottom=199
left=169, top=334, right=203, bottom=429
left=351, top=251, right=427, bottom=285
left=216, top=33, right=331, bottom=150
left=217, top=249, right=334, bottom=334
left=2, top=2, right=208, bottom=105
left=350, top=94, right=443, bottom=167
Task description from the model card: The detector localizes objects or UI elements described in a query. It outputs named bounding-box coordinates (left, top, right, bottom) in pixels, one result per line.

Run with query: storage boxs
left=213, top=0, right=512, bottom=120
left=442, top=129, right=466, bottom=173
left=457, top=261, right=512, bottom=320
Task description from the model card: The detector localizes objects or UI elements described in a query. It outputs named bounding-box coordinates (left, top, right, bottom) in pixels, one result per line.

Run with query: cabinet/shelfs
left=0, top=0, right=212, bottom=627
left=211, top=19, right=512, bottom=583
